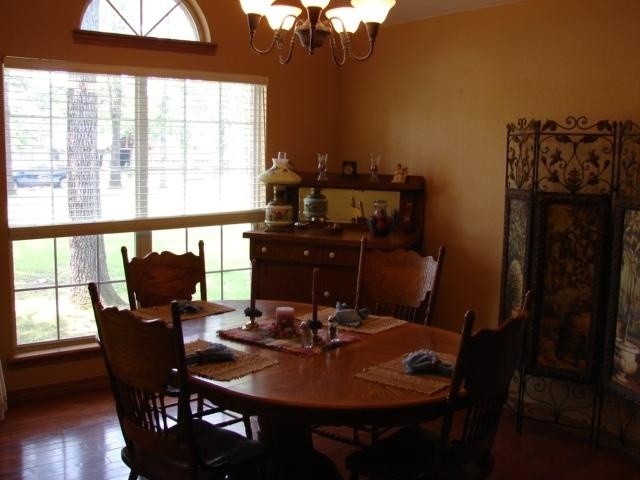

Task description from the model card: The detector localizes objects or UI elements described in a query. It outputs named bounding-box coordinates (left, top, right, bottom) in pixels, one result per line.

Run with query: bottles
left=328, top=314, right=338, bottom=342
left=370, top=200, right=391, bottom=238
left=300, top=321, right=314, bottom=349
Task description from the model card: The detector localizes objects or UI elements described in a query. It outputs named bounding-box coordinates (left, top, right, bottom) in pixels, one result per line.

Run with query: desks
left=243, top=167, right=427, bottom=313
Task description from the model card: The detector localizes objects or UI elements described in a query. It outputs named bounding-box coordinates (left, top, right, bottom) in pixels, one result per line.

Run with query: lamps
left=233, top=0, right=402, bottom=69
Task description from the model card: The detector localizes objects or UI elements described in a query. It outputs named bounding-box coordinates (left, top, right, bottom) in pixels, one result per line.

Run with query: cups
left=316, top=152, right=330, bottom=182
left=370, top=153, right=381, bottom=184
